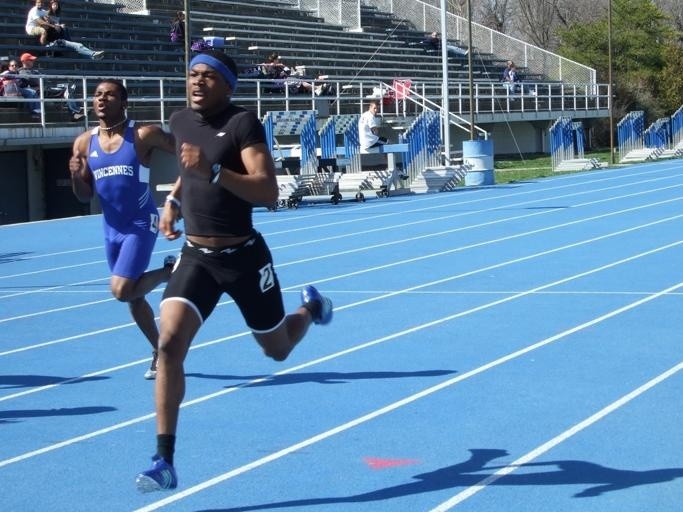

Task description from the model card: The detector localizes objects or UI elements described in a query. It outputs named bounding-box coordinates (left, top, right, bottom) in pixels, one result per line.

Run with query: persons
left=0, top=0, right=105, bottom=121
left=135, top=49, right=333, bottom=495
left=358, top=100, right=395, bottom=156
left=428, top=32, right=468, bottom=58
left=268, top=51, right=327, bottom=96
left=68, top=80, right=178, bottom=379
left=502, top=60, right=538, bottom=100
left=509, top=65, right=516, bottom=95
left=167, top=9, right=185, bottom=72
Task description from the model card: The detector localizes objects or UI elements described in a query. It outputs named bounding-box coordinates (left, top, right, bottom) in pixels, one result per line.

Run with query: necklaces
left=99, top=117, right=127, bottom=131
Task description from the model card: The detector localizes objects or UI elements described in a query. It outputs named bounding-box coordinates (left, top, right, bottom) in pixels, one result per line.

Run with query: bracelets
left=165, top=194, right=181, bottom=209
left=208, top=162, right=222, bottom=185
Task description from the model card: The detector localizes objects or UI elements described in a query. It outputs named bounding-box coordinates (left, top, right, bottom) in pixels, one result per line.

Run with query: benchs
left=0, top=0, right=186, bottom=111
left=189, top=1, right=442, bottom=112
left=447, top=38, right=593, bottom=109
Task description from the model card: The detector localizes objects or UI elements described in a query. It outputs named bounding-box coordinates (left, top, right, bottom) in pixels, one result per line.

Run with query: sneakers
left=143, top=348, right=158, bottom=379
left=134, top=453, right=178, bottom=494
left=300, top=284, right=334, bottom=326
left=163, top=256, right=176, bottom=267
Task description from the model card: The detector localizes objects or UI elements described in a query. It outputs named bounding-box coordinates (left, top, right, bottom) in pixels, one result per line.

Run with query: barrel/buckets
left=462, top=139, right=495, bottom=187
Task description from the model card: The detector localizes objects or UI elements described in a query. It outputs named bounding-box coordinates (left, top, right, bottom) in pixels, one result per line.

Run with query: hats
left=19, top=52, right=37, bottom=62
